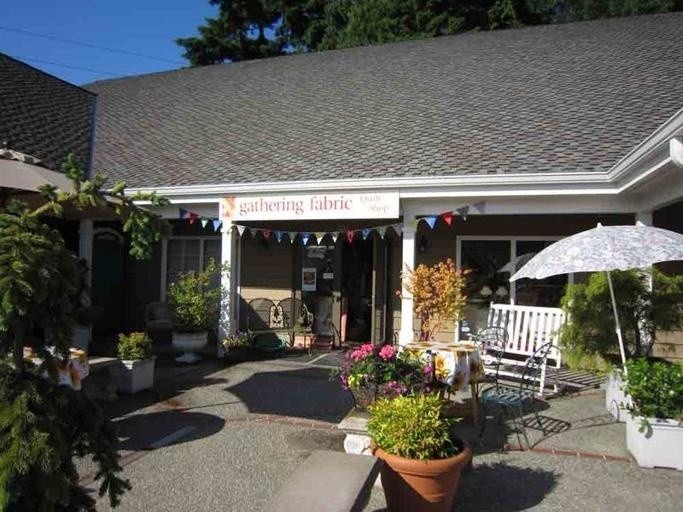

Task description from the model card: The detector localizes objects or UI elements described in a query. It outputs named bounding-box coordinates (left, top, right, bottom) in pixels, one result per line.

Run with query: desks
left=403, top=340, right=484, bottom=401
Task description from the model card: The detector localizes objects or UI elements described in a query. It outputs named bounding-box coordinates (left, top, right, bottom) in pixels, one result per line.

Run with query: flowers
left=325, top=341, right=448, bottom=397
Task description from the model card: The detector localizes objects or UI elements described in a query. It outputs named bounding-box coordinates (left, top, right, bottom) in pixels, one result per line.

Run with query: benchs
left=467, top=301, right=569, bottom=373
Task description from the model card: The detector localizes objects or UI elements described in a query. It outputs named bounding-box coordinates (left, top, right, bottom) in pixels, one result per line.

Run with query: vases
left=347, top=381, right=406, bottom=412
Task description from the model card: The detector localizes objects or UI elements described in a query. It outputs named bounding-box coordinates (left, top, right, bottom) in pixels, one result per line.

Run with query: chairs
left=472, top=326, right=554, bottom=452
left=245, top=296, right=314, bottom=355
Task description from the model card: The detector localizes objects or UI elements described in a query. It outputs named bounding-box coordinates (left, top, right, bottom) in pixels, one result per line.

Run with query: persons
left=312, top=289, right=332, bottom=333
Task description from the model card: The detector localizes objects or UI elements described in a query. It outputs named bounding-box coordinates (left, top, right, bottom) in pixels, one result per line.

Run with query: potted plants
left=364, top=392, right=472, bottom=511
left=112, top=330, right=157, bottom=393
left=558, top=267, right=682, bottom=471
left=166, top=257, right=233, bottom=363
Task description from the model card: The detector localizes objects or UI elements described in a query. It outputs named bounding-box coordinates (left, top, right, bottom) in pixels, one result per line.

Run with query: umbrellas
left=507, top=222, right=683, bottom=377
left=497, top=253, right=537, bottom=276
